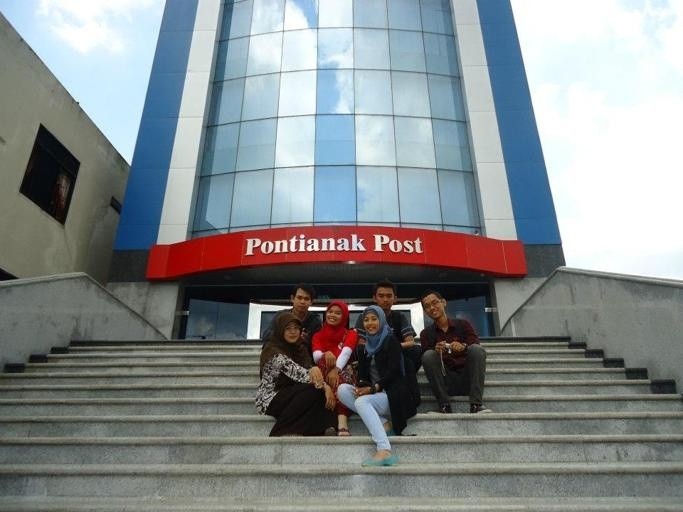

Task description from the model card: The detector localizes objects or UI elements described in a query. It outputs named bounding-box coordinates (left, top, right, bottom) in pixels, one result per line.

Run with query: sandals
left=338, top=428, right=351, bottom=435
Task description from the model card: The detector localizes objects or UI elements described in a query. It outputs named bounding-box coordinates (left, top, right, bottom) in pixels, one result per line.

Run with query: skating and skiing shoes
left=362, top=456, right=396, bottom=466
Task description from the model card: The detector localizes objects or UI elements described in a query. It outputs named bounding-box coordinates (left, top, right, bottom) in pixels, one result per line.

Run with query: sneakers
left=325, top=427, right=336, bottom=436
left=470, top=403, right=492, bottom=413
left=427, top=403, right=452, bottom=414
left=385, top=421, right=395, bottom=436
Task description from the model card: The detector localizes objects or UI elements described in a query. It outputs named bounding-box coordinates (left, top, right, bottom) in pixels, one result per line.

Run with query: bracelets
left=370, top=384, right=376, bottom=394
left=463, top=343, right=468, bottom=350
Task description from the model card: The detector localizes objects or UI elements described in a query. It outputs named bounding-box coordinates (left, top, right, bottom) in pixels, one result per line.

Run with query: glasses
left=425, top=299, right=441, bottom=310
left=285, top=324, right=301, bottom=330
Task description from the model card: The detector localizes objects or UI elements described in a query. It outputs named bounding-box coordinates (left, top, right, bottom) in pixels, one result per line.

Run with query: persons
left=310, top=298, right=361, bottom=438
left=354, top=281, right=425, bottom=376
left=416, top=290, right=492, bottom=413
left=338, top=306, right=421, bottom=469
left=262, top=283, right=323, bottom=361
left=253, top=311, right=336, bottom=437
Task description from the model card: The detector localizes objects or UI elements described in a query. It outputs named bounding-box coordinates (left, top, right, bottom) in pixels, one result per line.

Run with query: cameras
left=443, top=343, right=457, bottom=353
